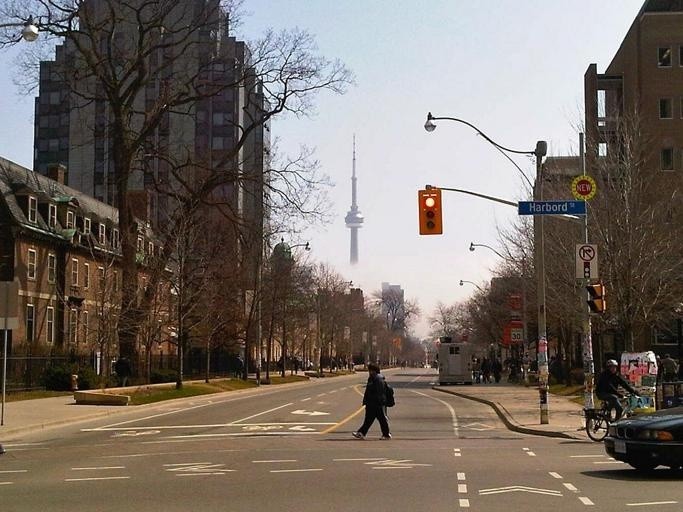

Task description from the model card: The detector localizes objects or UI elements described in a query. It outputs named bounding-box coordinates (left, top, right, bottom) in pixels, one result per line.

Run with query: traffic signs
left=517, top=200, right=585, bottom=215
left=510, top=327, right=522, bottom=341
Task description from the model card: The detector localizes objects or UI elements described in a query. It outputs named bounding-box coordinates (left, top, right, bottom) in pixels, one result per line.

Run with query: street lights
left=1, top=14, right=39, bottom=42
left=425, top=111, right=550, bottom=426
left=468, top=242, right=530, bottom=387
left=672, top=303, right=683, bottom=379
left=255, top=242, right=311, bottom=385
left=460, top=280, right=486, bottom=296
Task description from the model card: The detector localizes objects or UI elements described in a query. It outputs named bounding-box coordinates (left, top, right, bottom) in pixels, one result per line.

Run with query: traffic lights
left=585, top=284, right=607, bottom=316
left=419, top=188, right=443, bottom=235
left=584, top=262, right=591, bottom=277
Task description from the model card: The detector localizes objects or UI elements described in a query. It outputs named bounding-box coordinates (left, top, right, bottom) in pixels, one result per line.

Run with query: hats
left=605, top=359, right=618, bottom=367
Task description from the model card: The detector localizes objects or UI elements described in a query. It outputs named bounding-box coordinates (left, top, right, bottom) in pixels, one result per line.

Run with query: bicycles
left=581, top=390, right=635, bottom=442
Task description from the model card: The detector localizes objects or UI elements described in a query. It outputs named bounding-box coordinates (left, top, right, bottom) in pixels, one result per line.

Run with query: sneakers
left=352, top=431, right=368, bottom=440
left=379, top=434, right=392, bottom=441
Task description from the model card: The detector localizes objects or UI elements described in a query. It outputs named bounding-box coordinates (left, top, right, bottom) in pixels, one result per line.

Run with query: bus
left=436, top=342, right=471, bottom=386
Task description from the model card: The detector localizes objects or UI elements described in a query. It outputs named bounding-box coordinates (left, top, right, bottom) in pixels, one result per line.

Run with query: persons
left=292, top=357, right=299, bottom=374
left=593, top=359, right=640, bottom=422
left=232, top=354, right=243, bottom=379
left=620, top=359, right=655, bottom=407
left=351, top=362, right=394, bottom=441
left=676, top=383, right=683, bottom=396
left=326, top=356, right=353, bottom=371
left=278, top=356, right=283, bottom=374
left=660, top=353, right=678, bottom=382
left=472, top=352, right=531, bottom=384
left=655, top=355, right=664, bottom=381
left=114, top=355, right=131, bottom=386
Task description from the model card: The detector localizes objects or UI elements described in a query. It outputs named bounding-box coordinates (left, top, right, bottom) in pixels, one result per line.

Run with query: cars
left=321, top=355, right=343, bottom=370
left=276, top=354, right=312, bottom=370
left=604, top=405, right=682, bottom=478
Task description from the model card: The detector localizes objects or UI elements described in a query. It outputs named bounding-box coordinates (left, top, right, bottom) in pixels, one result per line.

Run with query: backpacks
left=384, top=376, right=395, bottom=407
